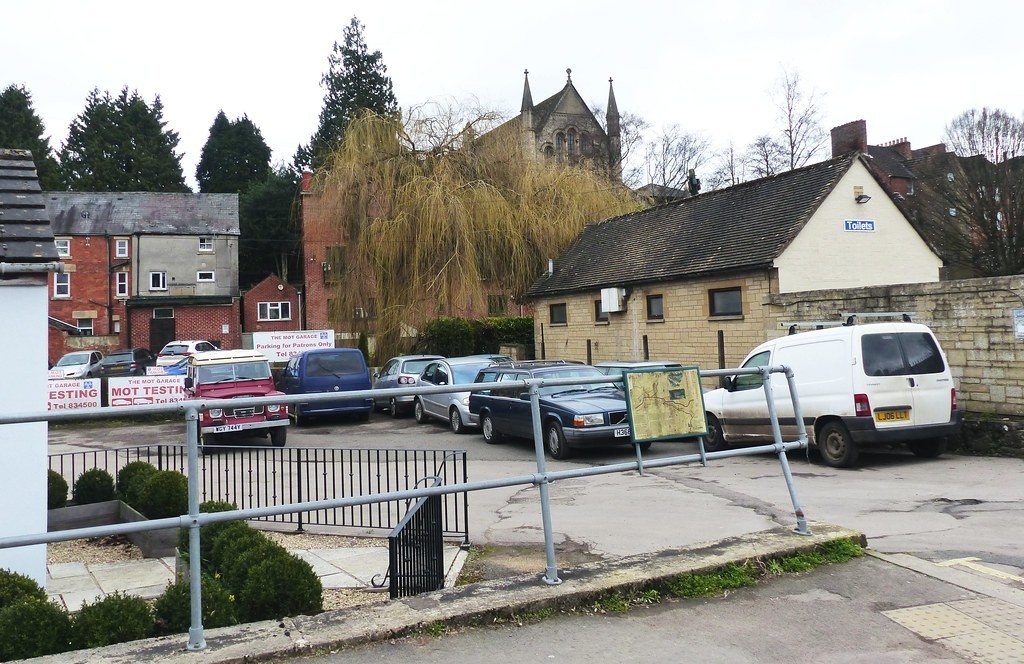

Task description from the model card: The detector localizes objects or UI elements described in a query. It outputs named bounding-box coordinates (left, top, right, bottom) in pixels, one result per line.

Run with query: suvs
left=468, top=359, right=652, bottom=459
left=184, top=349, right=290, bottom=454
left=702, top=311, right=958, bottom=469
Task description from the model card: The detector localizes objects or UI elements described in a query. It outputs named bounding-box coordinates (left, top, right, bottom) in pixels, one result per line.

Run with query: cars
left=161, top=357, right=225, bottom=375
left=595, top=361, right=714, bottom=402
left=371, top=355, right=448, bottom=419
left=413, top=357, right=515, bottom=435
left=464, top=354, right=516, bottom=364
left=100, top=346, right=158, bottom=379
left=49, top=351, right=105, bottom=379
left=156, top=340, right=220, bottom=368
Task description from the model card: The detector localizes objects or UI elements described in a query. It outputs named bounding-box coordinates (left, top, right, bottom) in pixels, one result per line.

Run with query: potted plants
left=48, top=460, right=324, bottom=626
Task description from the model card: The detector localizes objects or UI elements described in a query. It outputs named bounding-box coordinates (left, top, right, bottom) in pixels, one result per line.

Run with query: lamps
left=311, top=255, right=317, bottom=261
left=622, top=287, right=632, bottom=297
left=855, top=195, right=871, bottom=203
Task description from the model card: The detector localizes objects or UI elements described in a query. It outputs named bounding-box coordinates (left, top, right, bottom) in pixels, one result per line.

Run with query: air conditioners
left=351, top=307, right=369, bottom=319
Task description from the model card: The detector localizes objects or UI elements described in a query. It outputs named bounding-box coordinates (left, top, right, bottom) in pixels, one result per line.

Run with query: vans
left=281, top=348, right=373, bottom=427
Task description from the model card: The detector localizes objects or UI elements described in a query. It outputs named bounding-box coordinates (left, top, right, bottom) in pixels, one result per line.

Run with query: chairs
left=239, top=366, right=257, bottom=380
left=195, top=366, right=213, bottom=382
left=332, top=354, right=361, bottom=374
